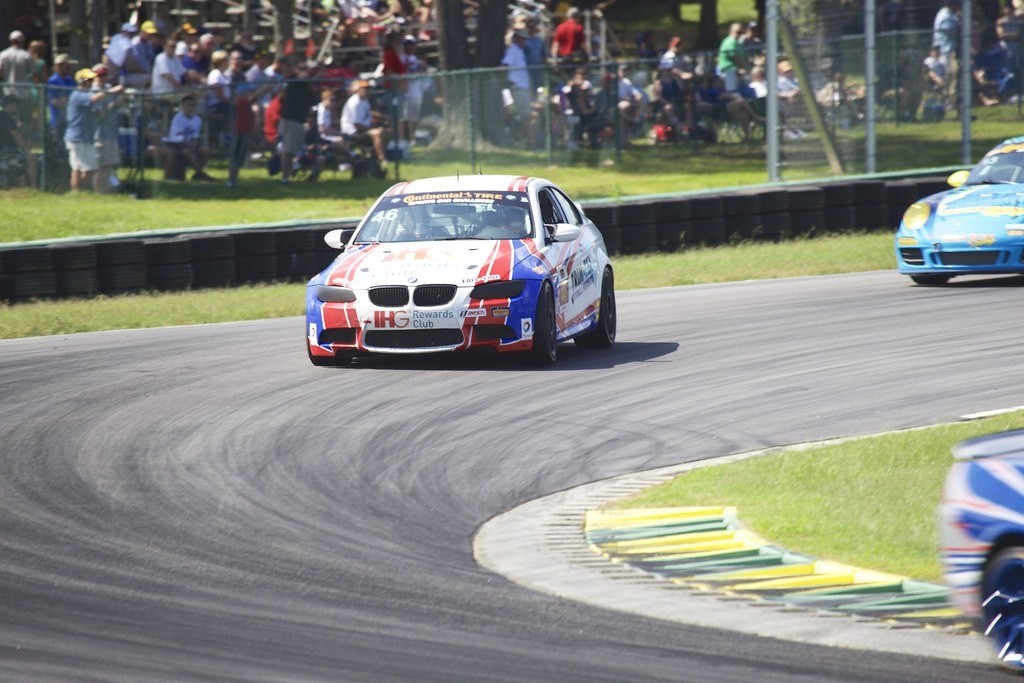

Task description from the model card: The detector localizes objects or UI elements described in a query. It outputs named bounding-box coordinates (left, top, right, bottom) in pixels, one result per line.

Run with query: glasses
left=235, top=57, right=246, bottom=61
left=328, top=98, right=338, bottom=102
left=736, top=31, right=744, bottom=35
left=98, top=74, right=109, bottom=79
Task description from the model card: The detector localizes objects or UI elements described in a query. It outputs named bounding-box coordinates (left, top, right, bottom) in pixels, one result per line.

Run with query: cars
left=894, top=136, right=1024, bottom=287
left=937, top=425, right=1024, bottom=673
left=303, top=173, right=618, bottom=368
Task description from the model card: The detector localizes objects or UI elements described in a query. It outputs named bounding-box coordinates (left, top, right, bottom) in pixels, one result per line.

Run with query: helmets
left=500, top=206, right=527, bottom=234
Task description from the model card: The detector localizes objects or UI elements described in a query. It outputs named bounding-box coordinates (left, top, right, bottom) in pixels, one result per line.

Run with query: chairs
left=778, top=95, right=816, bottom=130
left=478, top=210, right=498, bottom=227
left=726, top=97, right=767, bottom=148
left=396, top=205, right=428, bottom=228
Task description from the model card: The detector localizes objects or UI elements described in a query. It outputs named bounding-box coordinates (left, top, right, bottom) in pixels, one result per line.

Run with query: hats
left=122, top=23, right=138, bottom=32
left=255, top=48, right=275, bottom=60
left=402, top=35, right=419, bottom=45
left=92, top=63, right=110, bottom=75
left=747, top=20, right=760, bottom=31
left=672, top=36, right=685, bottom=48
left=777, top=59, right=792, bottom=72
left=141, top=20, right=158, bottom=34
left=349, top=79, right=372, bottom=93
left=182, top=23, right=197, bottom=34
left=513, top=23, right=529, bottom=38
left=54, top=53, right=79, bottom=64
left=75, top=68, right=97, bottom=82
left=30, top=40, right=47, bottom=46
left=566, top=7, right=579, bottom=17
left=10, top=30, right=25, bottom=41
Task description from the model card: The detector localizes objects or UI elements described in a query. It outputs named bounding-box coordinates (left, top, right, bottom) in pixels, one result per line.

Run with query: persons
left=0, top=0, right=438, bottom=179
left=502, top=0, right=1023, bottom=158
left=392, top=207, right=430, bottom=240
